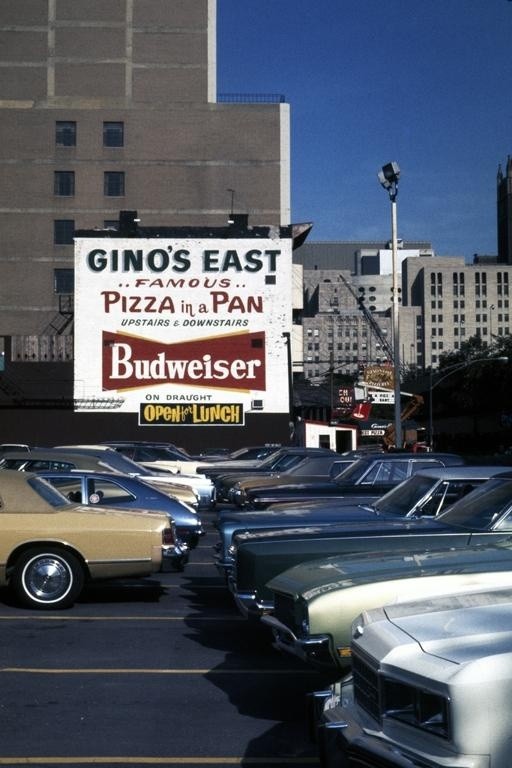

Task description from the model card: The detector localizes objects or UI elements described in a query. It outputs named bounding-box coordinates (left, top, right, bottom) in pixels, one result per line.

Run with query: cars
left=0, top=437, right=217, bottom=610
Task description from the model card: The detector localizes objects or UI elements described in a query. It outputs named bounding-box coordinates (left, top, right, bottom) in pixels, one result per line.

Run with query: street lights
left=375, top=161, right=404, bottom=449
